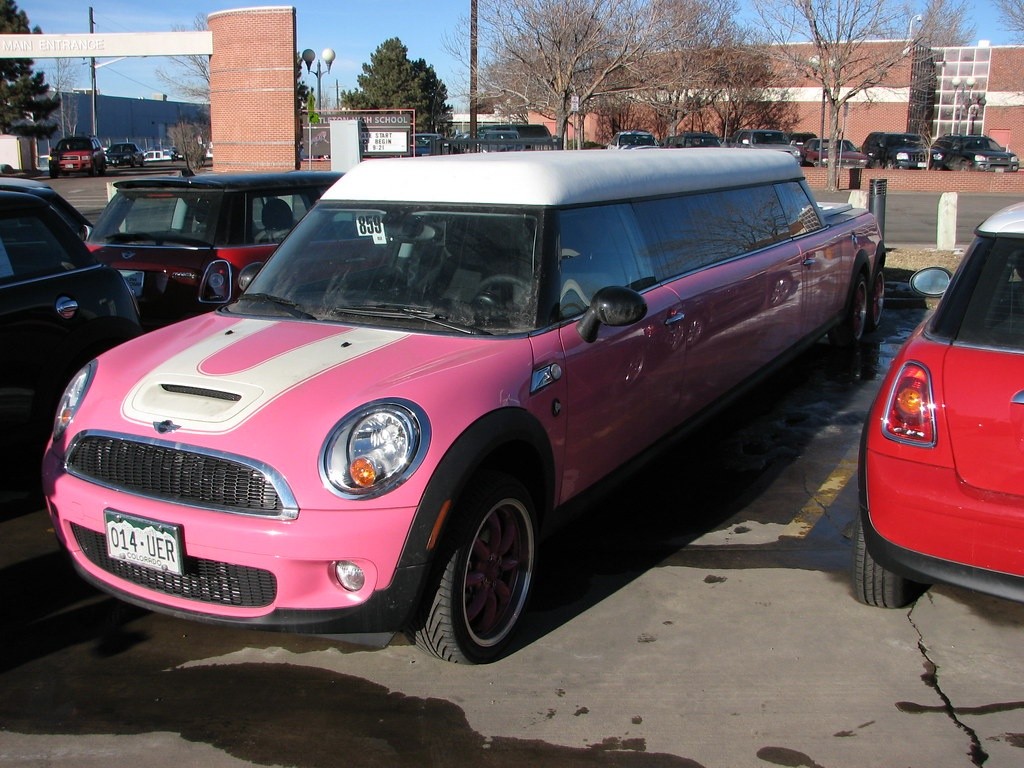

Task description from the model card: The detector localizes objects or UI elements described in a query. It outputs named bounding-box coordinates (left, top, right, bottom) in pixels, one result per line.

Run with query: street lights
left=809, top=53, right=838, bottom=168
left=909, top=13, right=922, bottom=59
left=301, top=47, right=336, bottom=113
left=952, top=76, right=977, bottom=134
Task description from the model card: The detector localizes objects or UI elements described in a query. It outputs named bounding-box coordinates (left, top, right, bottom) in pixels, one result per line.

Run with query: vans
left=47, top=134, right=107, bottom=178
left=41, top=150, right=882, bottom=663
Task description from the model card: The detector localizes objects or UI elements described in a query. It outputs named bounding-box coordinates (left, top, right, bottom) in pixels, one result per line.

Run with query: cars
left=410, top=123, right=560, bottom=157
left=855, top=200, right=1024, bottom=609
left=784, top=131, right=871, bottom=167
left=601, top=129, right=804, bottom=167
left=102, top=143, right=145, bottom=167
left=143, top=151, right=173, bottom=161
left=299, top=139, right=331, bottom=162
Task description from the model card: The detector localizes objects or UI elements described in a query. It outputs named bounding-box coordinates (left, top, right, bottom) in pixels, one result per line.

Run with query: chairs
left=261, top=199, right=293, bottom=243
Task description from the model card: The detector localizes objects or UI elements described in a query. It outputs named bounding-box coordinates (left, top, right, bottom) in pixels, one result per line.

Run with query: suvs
left=860, top=130, right=945, bottom=170
left=932, top=132, right=1020, bottom=173
left=0, top=175, right=343, bottom=455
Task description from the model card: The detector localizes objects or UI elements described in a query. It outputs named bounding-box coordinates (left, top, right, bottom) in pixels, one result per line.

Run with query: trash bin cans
left=848, top=168, right=862, bottom=189
left=48, top=160, right=60, bottom=179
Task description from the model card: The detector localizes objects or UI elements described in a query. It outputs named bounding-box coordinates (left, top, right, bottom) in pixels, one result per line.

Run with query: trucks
left=0, top=134, right=33, bottom=174
